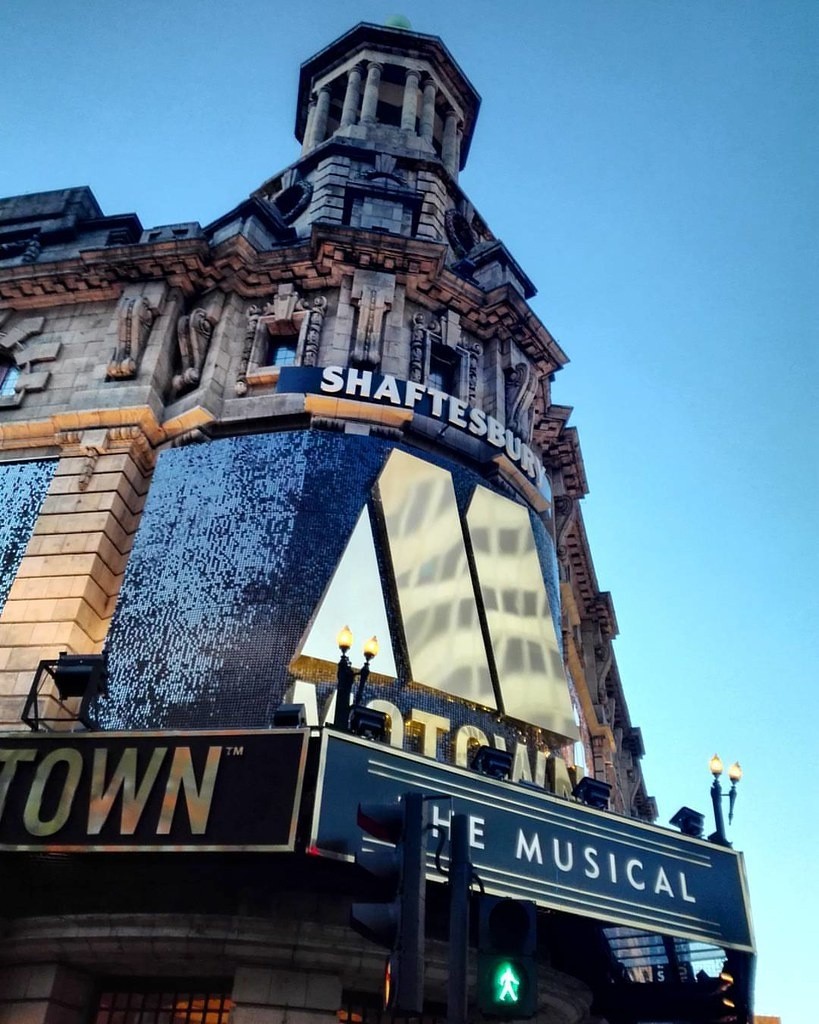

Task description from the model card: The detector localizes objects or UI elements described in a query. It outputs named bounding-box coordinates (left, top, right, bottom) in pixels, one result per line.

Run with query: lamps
left=324, top=624, right=379, bottom=737
left=348, top=704, right=386, bottom=742
left=706, top=754, right=742, bottom=846
left=571, top=776, right=613, bottom=810
left=470, top=745, right=515, bottom=781
left=268, top=703, right=308, bottom=728
left=669, top=807, right=705, bottom=836
left=18, top=654, right=111, bottom=733
